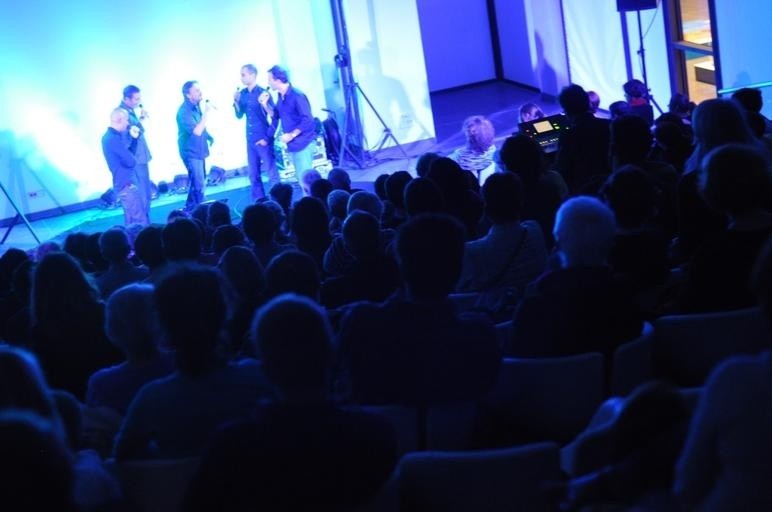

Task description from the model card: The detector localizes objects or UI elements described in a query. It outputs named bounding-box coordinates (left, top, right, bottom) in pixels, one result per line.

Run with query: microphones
left=206, top=100, right=216, bottom=109
left=139, top=104, right=149, bottom=118
left=261, top=86, right=270, bottom=101
left=235, top=87, right=239, bottom=102
left=127, top=125, right=142, bottom=134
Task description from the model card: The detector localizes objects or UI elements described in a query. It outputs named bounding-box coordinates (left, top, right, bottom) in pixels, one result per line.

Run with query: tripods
left=636, top=10, right=663, bottom=119
left=0, top=178, right=46, bottom=252
left=337, top=52, right=410, bottom=168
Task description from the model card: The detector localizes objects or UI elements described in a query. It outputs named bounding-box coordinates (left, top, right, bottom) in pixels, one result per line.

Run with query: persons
left=232, top=66, right=280, bottom=202
left=176, top=79, right=214, bottom=207
left=1, top=136, right=770, bottom=512
left=257, top=65, right=318, bottom=198
left=101, top=108, right=148, bottom=225
left=448, top=80, right=771, bottom=182
left=118, top=86, right=152, bottom=212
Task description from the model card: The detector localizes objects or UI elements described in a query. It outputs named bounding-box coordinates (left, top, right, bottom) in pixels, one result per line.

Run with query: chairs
left=103, top=287, right=771, bottom=512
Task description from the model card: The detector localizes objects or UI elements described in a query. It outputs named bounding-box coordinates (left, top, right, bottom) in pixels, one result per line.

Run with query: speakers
left=617, top=0, right=657, bottom=12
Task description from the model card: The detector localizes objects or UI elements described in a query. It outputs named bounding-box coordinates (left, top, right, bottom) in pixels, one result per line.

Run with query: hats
left=555, top=196, right=615, bottom=252
